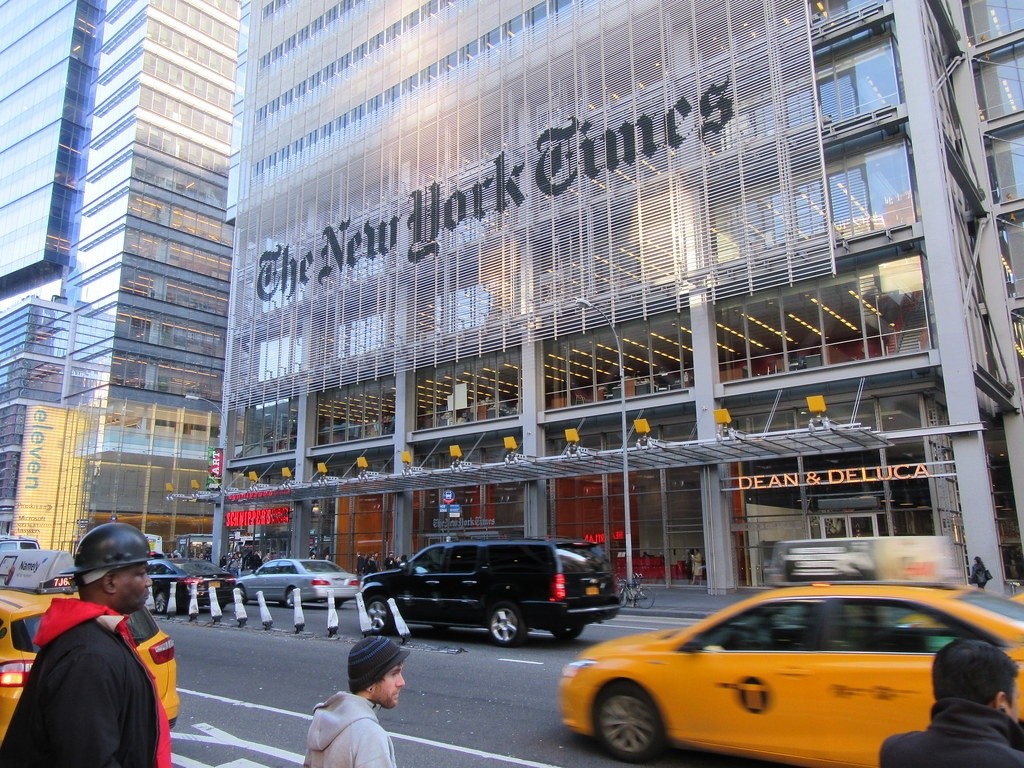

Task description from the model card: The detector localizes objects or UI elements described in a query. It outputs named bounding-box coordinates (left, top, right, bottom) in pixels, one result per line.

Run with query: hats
left=347, top=636, right=410, bottom=693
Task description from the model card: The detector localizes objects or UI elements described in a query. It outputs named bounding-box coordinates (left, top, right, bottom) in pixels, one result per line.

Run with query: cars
left=239, top=558, right=360, bottom=609
left=145, top=557, right=237, bottom=616
left=558, top=536, right=1024, bottom=768
left=0, top=587, right=180, bottom=748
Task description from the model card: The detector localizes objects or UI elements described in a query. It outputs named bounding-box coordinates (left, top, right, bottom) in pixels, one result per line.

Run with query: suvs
left=360, top=536, right=622, bottom=649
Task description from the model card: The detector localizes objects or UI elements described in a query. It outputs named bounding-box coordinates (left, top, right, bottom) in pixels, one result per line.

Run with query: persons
left=302, top=636, right=410, bottom=768
left=199, top=553, right=211, bottom=561
left=309, top=554, right=316, bottom=559
left=970, top=556, right=987, bottom=589
left=168, top=549, right=182, bottom=558
left=220, top=555, right=227, bottom=567
left=355, top=551, right=407, bottom=576
left=690, top=549, right=703, bottom=585
left=878, top=640, right=1024, bottom=768
left=233, top=552, right=239, bottom=560
left=0, top=522, right=172, bottom=768
left=249, top=550, right=270, bottom=569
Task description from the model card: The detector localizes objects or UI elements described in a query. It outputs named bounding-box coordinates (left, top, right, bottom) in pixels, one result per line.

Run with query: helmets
left=58, top=522, right=155, bottom=575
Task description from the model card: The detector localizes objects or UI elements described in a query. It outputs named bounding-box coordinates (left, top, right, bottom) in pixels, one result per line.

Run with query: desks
left=426, top=354, right=822, bottom=430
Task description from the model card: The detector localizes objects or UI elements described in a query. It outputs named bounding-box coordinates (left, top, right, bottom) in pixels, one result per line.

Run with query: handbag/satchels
left=985, top=570, right=993, bottom=581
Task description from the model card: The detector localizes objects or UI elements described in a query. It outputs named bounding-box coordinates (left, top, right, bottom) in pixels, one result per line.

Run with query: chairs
left=859, top=624, right=927, bottom=652
left=772, top=623, right=810, bottom=649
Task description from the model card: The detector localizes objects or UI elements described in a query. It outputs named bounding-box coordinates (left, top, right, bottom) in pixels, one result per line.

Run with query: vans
left=0, top=533, right=41, bottom=550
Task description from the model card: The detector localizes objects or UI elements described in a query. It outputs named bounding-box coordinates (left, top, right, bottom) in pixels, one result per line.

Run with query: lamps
left=317, top=462, right=328, bottom=476
left=504, top=436, right=518, bottom=452
left=400, top=451, right=412, bottom=465
left=713, top=408, right=732, bottom=430
left=357, top=456, right=368, bottom=471
left=282, top=467, right=293, bottom=480
left=565, top=427, right=580, bottom=446
left=191, top=479, right=200, bottom=491
left=633, top=419, right=651, bottom=438
left=449, top=444, right=464, bottom=460
left=808, top=395, right=827, bottom=418
left=165, top=483, right=174, bottom=493
left=249, top=470, right=259, bottom=482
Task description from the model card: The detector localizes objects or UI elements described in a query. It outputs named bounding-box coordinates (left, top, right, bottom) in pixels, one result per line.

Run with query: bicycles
left=614, top=572, right=656, bottom=609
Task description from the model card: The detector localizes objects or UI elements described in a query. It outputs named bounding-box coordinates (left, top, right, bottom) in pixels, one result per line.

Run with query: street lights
left=574, top=298, right=633, bottom=599
left=185, top=395, right=228, bottom=566
